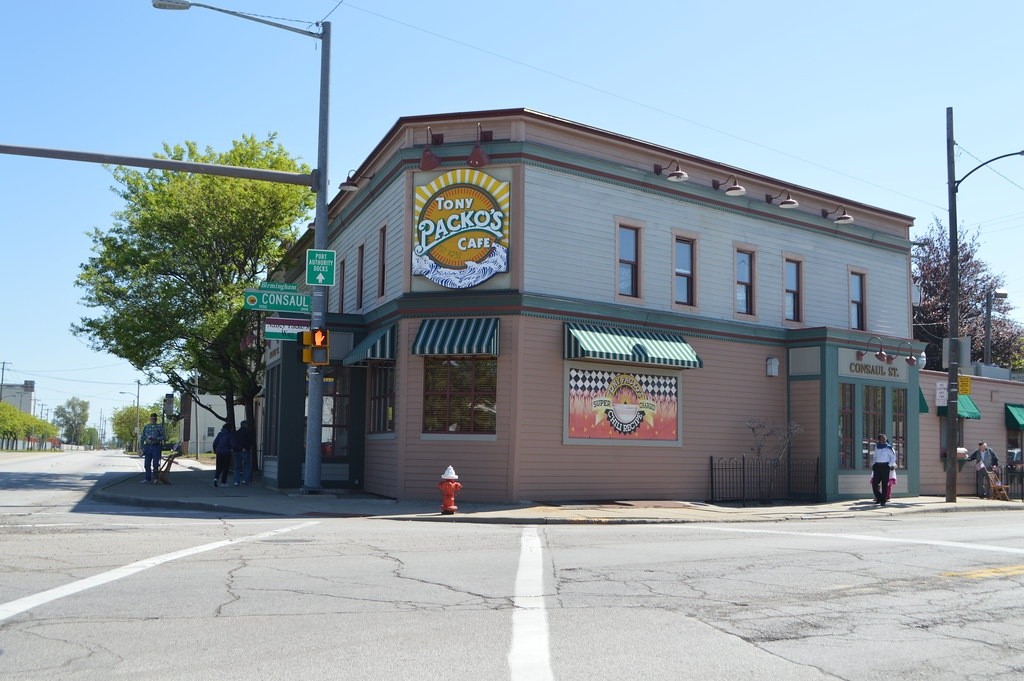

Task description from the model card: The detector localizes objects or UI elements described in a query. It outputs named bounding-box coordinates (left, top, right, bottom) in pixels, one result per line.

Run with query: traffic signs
left=305, top=246, right=336, bottom=286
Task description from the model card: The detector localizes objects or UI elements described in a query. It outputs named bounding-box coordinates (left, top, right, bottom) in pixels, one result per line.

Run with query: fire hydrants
left=436, top=464, right=463, bottom=516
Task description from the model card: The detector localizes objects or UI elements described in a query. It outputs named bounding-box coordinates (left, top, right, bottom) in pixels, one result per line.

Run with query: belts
left=146, top=443, right=159, bottom=445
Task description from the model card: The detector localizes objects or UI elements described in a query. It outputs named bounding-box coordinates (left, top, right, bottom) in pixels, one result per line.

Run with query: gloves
left=139, top=449, right=143, bottom=457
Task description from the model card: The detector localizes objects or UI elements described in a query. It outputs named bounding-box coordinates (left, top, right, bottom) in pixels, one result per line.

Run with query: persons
left=139, top=413, right=165, bottom=484
left=968, top=441, right=999, bottom=500
left=871, top=433, right=896, bottom=505
left=212, top=420, right=253, bottom=487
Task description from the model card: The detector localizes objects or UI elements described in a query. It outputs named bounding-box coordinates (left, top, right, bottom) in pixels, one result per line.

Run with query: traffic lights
left=297, top=332, right=311, bottom=363
left=310, top=328, right=329, bottom=365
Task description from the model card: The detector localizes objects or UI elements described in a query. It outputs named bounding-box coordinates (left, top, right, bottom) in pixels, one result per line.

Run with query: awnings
left=919, top=384, right=1024, bottom=429
left=411, top=317, right=500, bottom=357
left=562, top=322, right=703, bottom=371
left=342, top=324, right=398, bottom=367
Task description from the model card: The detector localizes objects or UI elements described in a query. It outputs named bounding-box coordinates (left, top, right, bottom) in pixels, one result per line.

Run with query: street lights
left=947, top=102, right=1023, bottom=507
left=151, top=0, right=332, bottom=490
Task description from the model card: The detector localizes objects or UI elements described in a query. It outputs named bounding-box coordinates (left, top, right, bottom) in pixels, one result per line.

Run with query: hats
left=150, top=413, right=157, bottom=418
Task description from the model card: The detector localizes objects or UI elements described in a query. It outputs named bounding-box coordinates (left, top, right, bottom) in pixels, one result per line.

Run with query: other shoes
left=235, top=483, right=239, bottom=486
left=221, top=483, right=229, bottom=488
left=213, top=479, right=219, bottom=487
left=877, top=498, right=890, bottom=506
left=140, top=480, right=152, bottom=484
left=242, top=481, right=249, bottom=486
left=154, top=479, right=159, bottom=484
left=981, top=496, right=984, bottom=499
